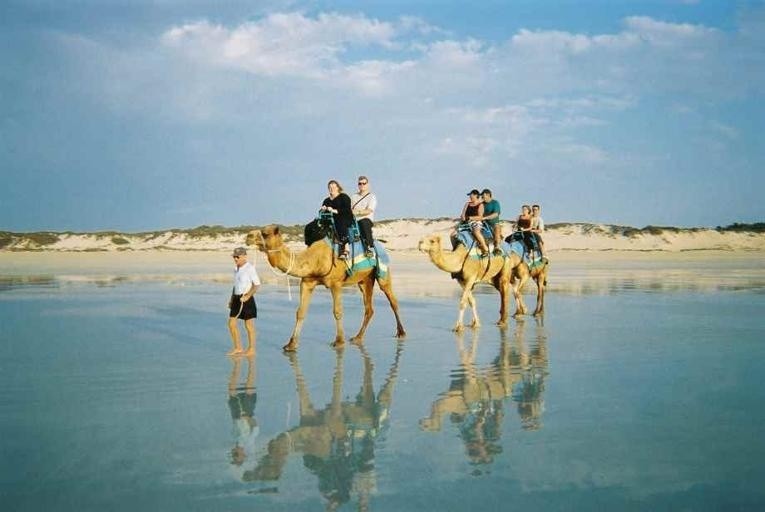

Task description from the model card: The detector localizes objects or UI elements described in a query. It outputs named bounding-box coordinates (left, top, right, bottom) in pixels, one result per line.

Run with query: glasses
left=234, top=256, right=242, bottom=259
left=358, top=183, right=366, bottom=185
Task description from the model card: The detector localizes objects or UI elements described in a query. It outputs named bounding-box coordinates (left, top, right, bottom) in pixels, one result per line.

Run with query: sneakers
left=481, top=248, right=502, bottom=257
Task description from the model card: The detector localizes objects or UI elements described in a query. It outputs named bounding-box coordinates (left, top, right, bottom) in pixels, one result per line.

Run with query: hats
left=230, top=247, right=246, bottom=256
left=467, top=189, right=490, bottom=195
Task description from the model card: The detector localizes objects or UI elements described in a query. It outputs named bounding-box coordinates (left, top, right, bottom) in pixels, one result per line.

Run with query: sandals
left=366, top=249, right=373, bottom=257
left=339, top=251, right=351, bottom=258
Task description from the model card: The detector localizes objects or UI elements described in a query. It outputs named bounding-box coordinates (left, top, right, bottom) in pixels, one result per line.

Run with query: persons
left=350, top=176, right=376, bottom=257
left=457, top=377, right=545, bottom=463
left=505, top=205, right=536, bottom=258
left=319, top=436, right=354, bottom=512
left=450, top=190, right=489, bottom=258
left=481, top=188, right=502, bottom=255
left=354, top=432, right=377, bottom=512
left=530, top=205, right=546, bottom=258
left=319, top=180, right=352, bottom=259
left=227, top=356, right=259, bottom=464
left=225, top=248, right=260, bottom=356
